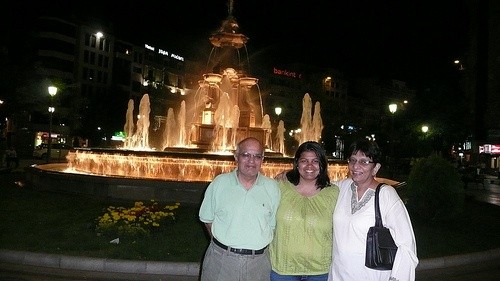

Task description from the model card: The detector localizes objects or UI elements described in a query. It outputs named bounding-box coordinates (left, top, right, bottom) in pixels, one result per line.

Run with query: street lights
left=388, top=102, right=398, bottom=160
left=47, top=84, right=59, bottom=159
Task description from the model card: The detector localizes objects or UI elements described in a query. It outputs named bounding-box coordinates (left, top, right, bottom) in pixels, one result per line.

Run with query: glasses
left=237, top=152, right=264, bottom=160
left=347, top=159, right=374, bottom=165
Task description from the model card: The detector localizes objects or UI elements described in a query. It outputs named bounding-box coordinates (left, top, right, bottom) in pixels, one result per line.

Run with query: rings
left=281, top=172, right=283, bottom=174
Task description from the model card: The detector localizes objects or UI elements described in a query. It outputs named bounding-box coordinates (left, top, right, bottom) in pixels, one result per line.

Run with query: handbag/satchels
left=365, top=183, right=409, bottom=270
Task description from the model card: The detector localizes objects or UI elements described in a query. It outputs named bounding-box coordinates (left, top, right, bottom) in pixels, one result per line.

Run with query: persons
left=270, top=141, right=339, bottom=281
left=274, top=138, right=419, bottom=281
left=198, top=137, right=281, bottom=281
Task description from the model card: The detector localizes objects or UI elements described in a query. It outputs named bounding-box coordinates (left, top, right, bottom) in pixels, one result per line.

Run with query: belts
left=211, top=236, right=267, bottom=255
